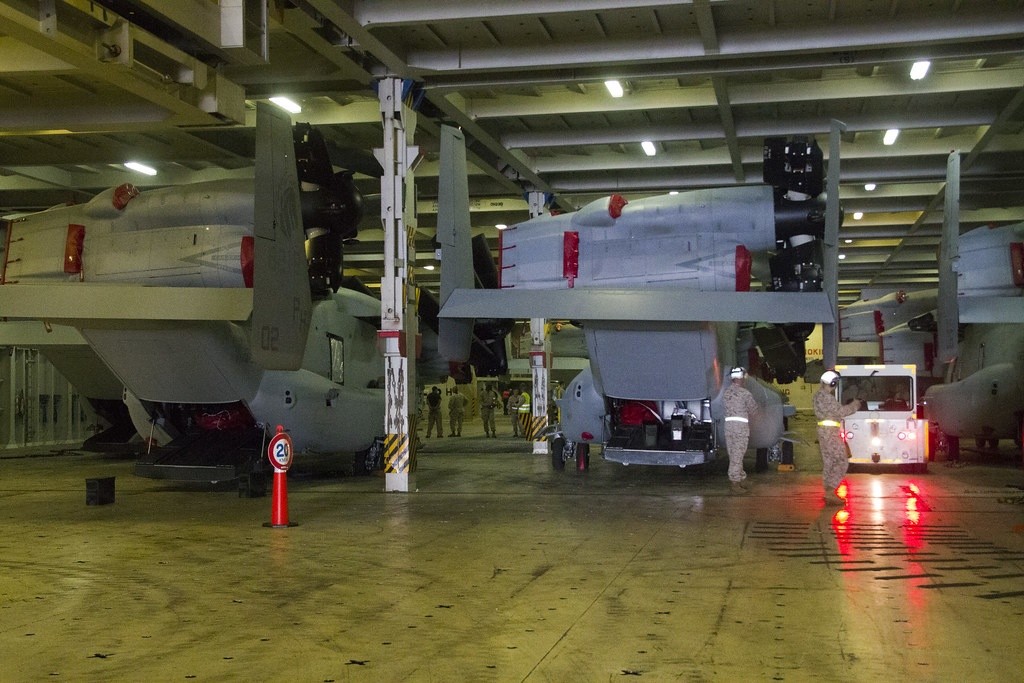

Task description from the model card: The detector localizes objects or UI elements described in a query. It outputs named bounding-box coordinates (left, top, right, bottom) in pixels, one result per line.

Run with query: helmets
left=519, top=383, right=526, bottom=388
left=820, top=371, right=840, bottom=384
left=485, top=381, right=492, bottom=386
left=731, top=367, right=748, bottom=379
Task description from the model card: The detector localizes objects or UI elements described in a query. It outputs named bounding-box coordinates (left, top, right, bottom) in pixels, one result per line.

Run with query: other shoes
left=426, top=435, right=431, bottom=438
left=437, top=435, right=444, bottom=438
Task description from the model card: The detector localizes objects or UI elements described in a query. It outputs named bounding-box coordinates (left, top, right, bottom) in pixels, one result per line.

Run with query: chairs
left=846, top=398, right=868, bottom=411
left=885, top=398, right=908, bottom=410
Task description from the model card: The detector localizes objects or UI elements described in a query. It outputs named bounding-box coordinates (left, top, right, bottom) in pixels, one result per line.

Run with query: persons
left=503, top=384, right=530, bottom=438
left=426, top=386, right=444, bottom=438
left=447, top=386, right=469, bottom=437
left=724, top=367, right=758, bottom=480
left=479, top=382, right=497, bottom=438
left=552, top=381, right=566, bottom=407
left=813, top=371, right=861, bottom=503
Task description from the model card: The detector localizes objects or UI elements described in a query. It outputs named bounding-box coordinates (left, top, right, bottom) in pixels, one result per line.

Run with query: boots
left=456, top=433, right=461, bottom=437
left=485, top=431, right=490, bottom=438
left=823, top=484, right=846, bottom=506
left=492, top=431, right=496, bottom=438
left=447, top=431, right=455, bottom=437
left=729, top=481, right=748, bottom=494
